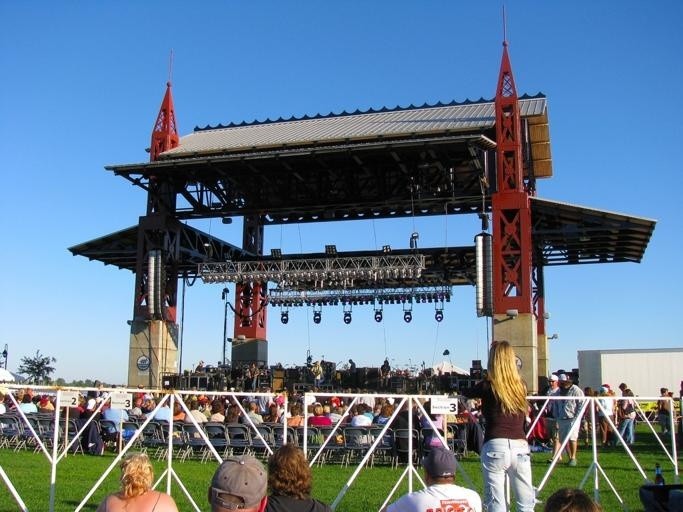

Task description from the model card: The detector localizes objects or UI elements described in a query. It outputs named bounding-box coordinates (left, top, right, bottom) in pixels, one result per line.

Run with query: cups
left=549, top=380, right=555, bottom=383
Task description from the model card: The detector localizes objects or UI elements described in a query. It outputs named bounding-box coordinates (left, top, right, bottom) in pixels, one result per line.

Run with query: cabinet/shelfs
left=652, top=462, right=667, bottom=486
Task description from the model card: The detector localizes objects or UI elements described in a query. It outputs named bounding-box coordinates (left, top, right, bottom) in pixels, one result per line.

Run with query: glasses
left=270, top=292, right=450, bottom=322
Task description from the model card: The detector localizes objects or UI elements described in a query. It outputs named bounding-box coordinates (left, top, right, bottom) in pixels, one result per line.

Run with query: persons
left=658, top=388, right=676, bottom=442
left=379, top=446, right=482, bottom=511
left=525, top=373, right=636, bottom=467
left=311, top=362, right=322, bottom=390
left=3, top=386, right=484, bottom=462
left=94, top=452, right=181, bottom=511
left=195, top=362, right=204, bottom=373
left=250, top=363, right=259, bottom=391
left=259, top=442, right=333, bottom=512
left=543, top=486, right=603, bottom=512
left=205, top=454, right=269, bottom=512
left=381, top=361, right=390, bottom=392
left=461, top=339, right=537, bottom=511
left=349, top=361, right=356, bottom=384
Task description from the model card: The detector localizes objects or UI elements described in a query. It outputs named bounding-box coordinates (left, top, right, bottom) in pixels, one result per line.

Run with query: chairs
left=568, top=459, right=576, bottom=467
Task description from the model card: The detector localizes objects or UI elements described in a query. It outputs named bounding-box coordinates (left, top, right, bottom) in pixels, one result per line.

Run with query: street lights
left=623, top=404, right=634, bottom=414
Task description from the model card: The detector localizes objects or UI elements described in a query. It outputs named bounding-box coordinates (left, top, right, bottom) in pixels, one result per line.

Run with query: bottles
left=602, top=384, right=610, bottom=388
left=600, top=387, right=608, bottom=393
left=87, top=398, right=96, bottom=409
left=559, top=374, right=567, bottom=381
left=210, top=454, right=268, bottom=510
left=423, top=448, right=457, bottom=477
left=4, top=394, right=57, bottom=407
left=549, top=375, right=558, bottom=381
left=640, top=483, right=683, bottom=512
